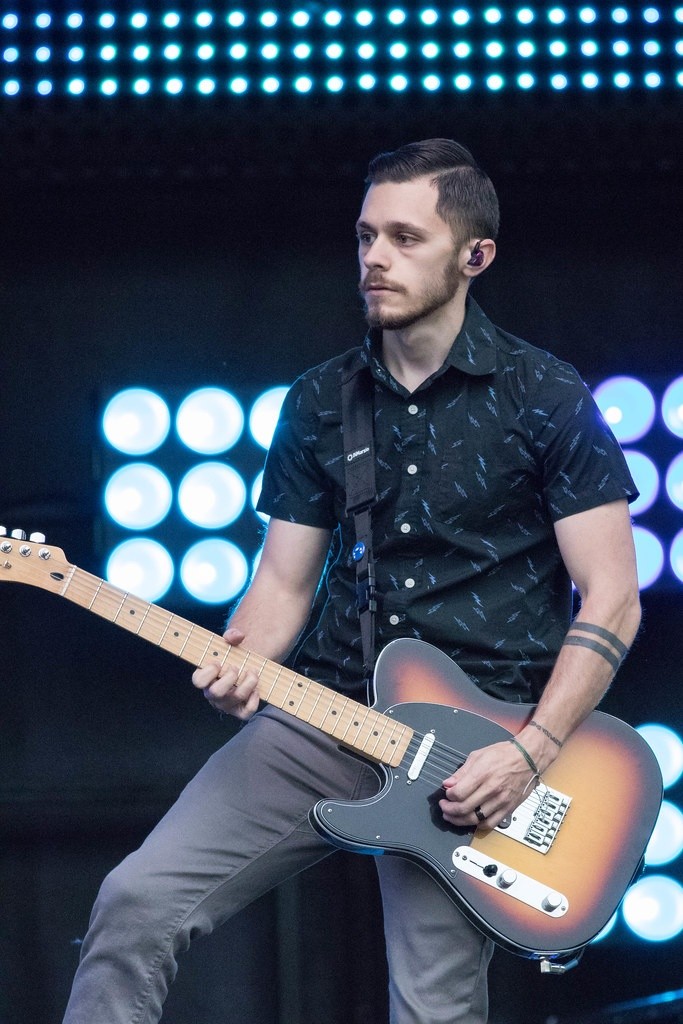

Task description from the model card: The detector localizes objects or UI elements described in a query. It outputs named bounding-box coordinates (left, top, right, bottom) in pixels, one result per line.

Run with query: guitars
left=5, top=522, right=667, bottom=963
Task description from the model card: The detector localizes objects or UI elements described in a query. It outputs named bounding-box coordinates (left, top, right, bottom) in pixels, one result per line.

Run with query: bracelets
left=508, top=738, right=549, bottom=821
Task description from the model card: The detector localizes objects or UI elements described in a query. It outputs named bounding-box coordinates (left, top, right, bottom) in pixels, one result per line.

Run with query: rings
left=474, top=806, right=487, bottom=820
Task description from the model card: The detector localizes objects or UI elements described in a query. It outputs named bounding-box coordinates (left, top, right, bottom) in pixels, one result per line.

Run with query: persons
left=61, top=142, right=643, bottom=1023
